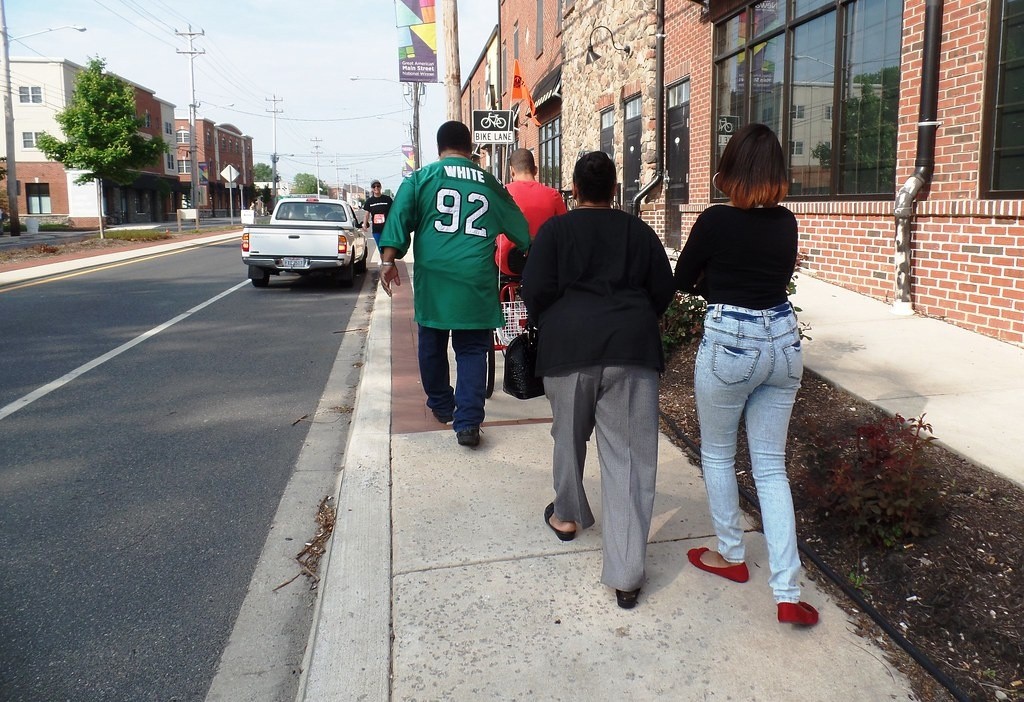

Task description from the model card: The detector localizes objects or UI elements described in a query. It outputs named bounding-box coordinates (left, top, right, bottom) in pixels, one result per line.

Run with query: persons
left=492, top=148, right=567, bottom=314
left=290, top=205, right=334, bottom=220
left=520, top=152, right=675, bottom=609
left=379, top=121, right=532, bottom=448
left=362, top=180, right=395, bottom=265
left=675, top=123, right=819, bottom=625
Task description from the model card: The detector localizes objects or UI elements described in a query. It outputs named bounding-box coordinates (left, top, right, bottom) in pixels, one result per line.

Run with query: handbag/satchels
left=503, top=332, right=545, bottom=400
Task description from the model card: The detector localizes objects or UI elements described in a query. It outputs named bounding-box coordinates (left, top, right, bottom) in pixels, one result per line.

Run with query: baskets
left=495, top=301, right=529, bottom=346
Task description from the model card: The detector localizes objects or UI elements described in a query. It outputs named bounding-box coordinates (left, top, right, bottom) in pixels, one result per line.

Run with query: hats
left=371, top=180, right=381, bottom=188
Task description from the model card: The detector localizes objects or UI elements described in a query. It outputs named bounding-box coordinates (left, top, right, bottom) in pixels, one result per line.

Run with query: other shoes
left=433, top=411, right=454, bottom=423
left=688, top=548, right=749, bottom=583
left=457, top=428, right=480, bottom=446
left=778, top=601, right=819, bottom=625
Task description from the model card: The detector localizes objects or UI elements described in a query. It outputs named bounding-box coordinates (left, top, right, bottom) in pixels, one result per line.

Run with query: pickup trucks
left=241, top=197, right=370, bottom=289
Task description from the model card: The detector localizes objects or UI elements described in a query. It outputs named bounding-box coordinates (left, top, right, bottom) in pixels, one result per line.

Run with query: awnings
left=526, top=62, right=562, bottom=118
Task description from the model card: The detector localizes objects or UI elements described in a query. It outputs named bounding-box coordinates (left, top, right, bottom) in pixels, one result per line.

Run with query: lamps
left=585, top=25, right=632, bottom=64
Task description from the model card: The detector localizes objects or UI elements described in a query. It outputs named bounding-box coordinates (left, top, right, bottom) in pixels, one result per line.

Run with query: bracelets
left=383, top=261, right=395, bottom=266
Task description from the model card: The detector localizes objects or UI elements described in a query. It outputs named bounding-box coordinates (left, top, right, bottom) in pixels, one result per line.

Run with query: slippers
left=545, top=503, right=576, bottom=540
left=615, top=588, right=640, bottom=609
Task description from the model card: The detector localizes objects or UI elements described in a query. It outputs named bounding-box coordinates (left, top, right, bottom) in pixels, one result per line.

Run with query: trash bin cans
left=27, top=218, right=39, bottom=232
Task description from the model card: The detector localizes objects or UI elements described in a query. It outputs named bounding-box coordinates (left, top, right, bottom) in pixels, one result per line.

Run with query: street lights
left=190, top=103, right=235, bottom=223
left=351, top=76, right=420, bottom=171
left=0, top=25, right=87, bottom=237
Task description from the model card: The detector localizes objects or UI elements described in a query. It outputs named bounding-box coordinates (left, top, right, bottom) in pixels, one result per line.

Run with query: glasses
left=373, top=186, right=379, bottom=188
